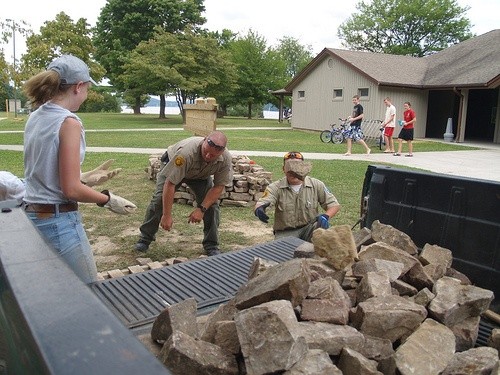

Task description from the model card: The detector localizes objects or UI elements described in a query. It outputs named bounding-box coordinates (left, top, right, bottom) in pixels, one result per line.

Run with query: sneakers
left=136, top=238, right=151, bottom=250
left=206, top=249, right=219, bottom=256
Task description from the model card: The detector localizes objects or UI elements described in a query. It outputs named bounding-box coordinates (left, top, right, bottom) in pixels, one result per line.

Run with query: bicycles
left=320, top=118, right=348, bottom=143
left=331, top=119, right=350, bottom=145
left=376, top=119, right=386, bottom=150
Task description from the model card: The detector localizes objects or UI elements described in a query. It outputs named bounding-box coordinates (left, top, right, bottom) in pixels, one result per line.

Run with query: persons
left=380, top=97, right=397, bottom=153
left=392, top=102, right=416, bottom=157
left=24, top=55, right=138, bottom=283
left=136, top=130, right=232, bottom=256
left=344, top=95, right=371, bottom=156
left=254, top=151, right=340, bottom=244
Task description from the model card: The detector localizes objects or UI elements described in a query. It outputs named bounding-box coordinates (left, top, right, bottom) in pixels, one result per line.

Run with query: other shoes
left=394, top=153, right=400, bottom=156
left=405, top=153, right=413, bottom=157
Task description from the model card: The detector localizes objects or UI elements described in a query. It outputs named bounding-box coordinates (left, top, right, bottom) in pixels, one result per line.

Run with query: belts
left=23, top=202, right=78, bottom=212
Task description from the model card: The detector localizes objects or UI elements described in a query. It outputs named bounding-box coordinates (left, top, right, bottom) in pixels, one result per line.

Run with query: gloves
left=79, top=158, right=123, bottom=188
left=311, top=214, right=329, bottom=229
left=255, top=202, right=271, bottom=223
left=97, top=190, right=138, bottom=216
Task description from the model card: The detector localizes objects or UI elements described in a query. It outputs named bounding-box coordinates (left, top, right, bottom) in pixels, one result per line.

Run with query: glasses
left=284, top=152, right=304, bottom=159
left=207, top=138, right=225, bottom=151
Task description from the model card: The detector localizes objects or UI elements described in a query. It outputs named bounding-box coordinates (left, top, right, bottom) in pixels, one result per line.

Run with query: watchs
left=197, top=206, right=207, bottom=213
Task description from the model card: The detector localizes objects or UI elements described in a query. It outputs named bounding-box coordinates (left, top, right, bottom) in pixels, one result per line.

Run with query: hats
left=47, top=54, right=99, bottom=88
